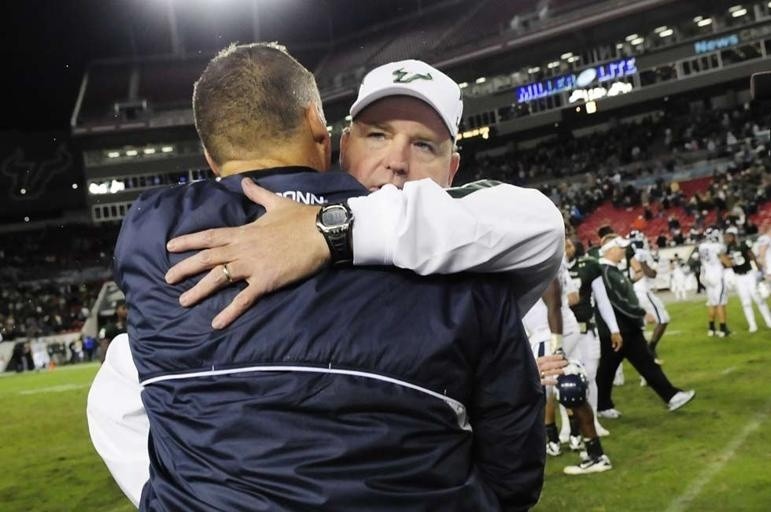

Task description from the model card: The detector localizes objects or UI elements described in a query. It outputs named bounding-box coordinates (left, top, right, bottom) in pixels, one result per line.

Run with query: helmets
left=627, top=229, right=645, bottom=244
left=553, top=361, right=591, bottom=408
left=703, top=225, right=720, bottom=238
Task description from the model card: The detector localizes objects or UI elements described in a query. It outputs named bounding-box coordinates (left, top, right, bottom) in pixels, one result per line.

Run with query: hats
left=598, top=234, right=632, bottom=255
left=348, top=59, right=463, bottom=136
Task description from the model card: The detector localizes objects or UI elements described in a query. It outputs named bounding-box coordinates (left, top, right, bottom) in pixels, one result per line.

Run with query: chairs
left=573, top=176, right=771, bottom=253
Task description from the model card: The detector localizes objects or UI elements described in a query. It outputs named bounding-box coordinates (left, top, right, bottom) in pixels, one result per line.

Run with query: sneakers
left=612, top=372, right=625, bottom=386
left=563, top=453, right=612, bottom=476
left=593, top=420, right=611, bottom=437
left=559, top=421, right=570, bottom=443
left=568, top=434, right=584, bottom=452
left=546, top=440, right=561, bottom=456
left=717, top=330, right=737, bottom=339
left=707, top=329, right=717, bottom=337
left=748, top=322, right=758, bottom=333
left=667, top=389, right=697, bottom=414
left=596, top=405, right=621, bottom=419
left=652, top=352, right=665, bottom=366
